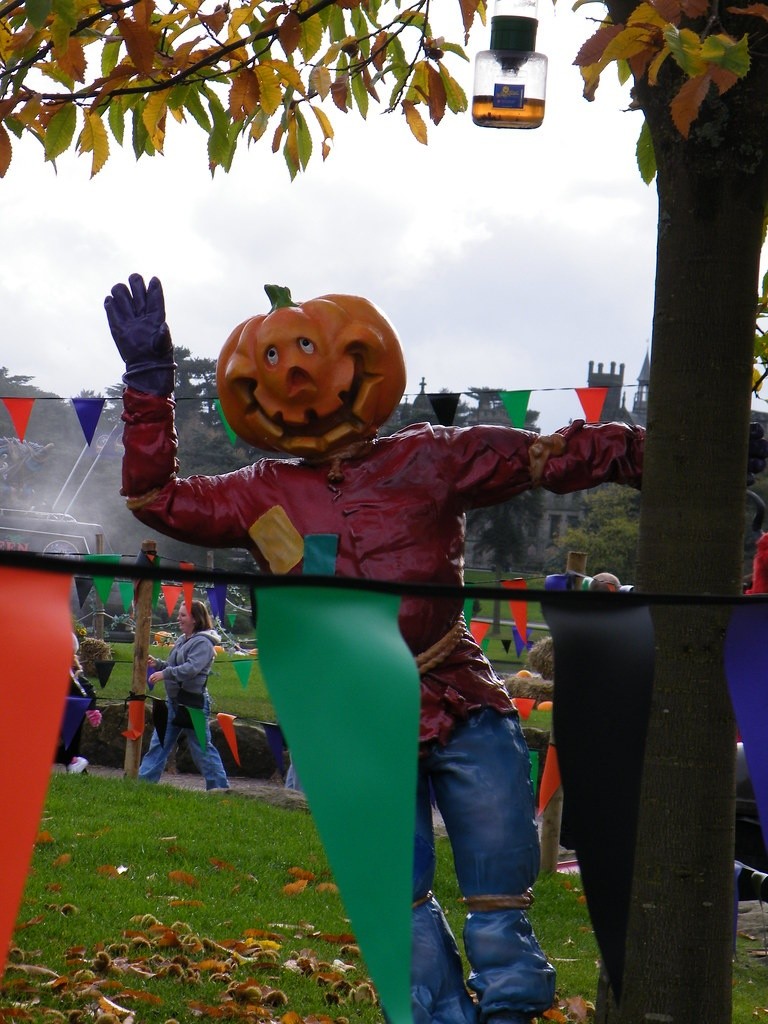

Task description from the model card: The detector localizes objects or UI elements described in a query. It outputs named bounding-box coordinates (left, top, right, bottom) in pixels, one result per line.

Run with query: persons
left=64, top=632, right=101, bottom=777
left=104, top=271, right=768, bottom=1024
left=139, top=600, right=230, bottom=791
left=285, top=751, right=304, bottom=792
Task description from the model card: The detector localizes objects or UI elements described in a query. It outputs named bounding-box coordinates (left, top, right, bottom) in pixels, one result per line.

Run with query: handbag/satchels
left=176, top=688, right=205, bottom=710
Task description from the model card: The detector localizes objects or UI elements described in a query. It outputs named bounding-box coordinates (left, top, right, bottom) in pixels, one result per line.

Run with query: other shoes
left=65, top=757, right=89, bottom=774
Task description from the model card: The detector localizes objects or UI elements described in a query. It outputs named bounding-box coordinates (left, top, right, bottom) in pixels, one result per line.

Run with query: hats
left=72, top=632, right=79, bottom=656
